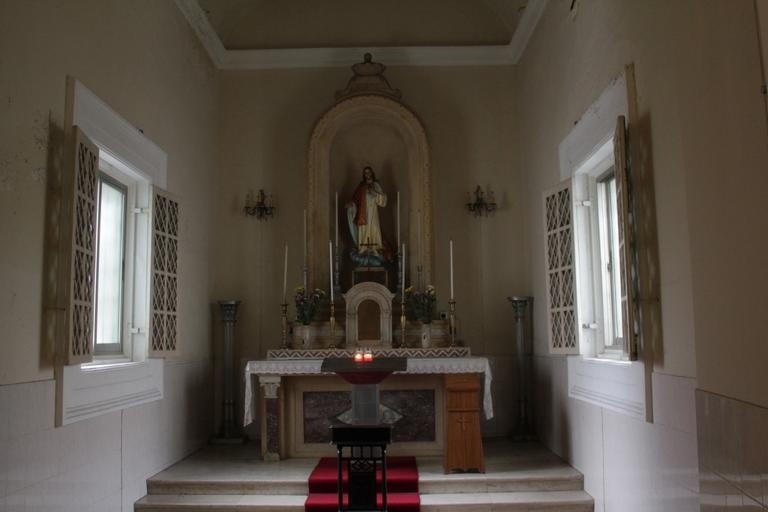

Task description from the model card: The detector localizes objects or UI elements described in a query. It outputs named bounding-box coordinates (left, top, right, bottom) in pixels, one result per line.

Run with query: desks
left=329, top=424, right=390, bottom=512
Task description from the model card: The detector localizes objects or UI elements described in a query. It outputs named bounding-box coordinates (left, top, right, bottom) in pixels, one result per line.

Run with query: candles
left=397, top=191, right=402, bottom=254
left=334, top=191, right=339, bottom=248
left=303, top=208, right=307, bottom=267
left=282, top=243, right=288, bottom=304
left=401, top=243, right=405, bottom=304
left=328, top=239, right=333, bottom=302
left=450, top=239, right=454, bottom=300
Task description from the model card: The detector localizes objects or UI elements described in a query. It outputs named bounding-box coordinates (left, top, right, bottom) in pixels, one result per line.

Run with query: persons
left=344, top=167, right=387, bottom=257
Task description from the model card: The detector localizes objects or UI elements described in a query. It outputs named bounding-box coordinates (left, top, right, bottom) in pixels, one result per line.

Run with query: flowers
left=405, top=285, right=435, bottom=325
left=294, top=286, right=325, bottom=326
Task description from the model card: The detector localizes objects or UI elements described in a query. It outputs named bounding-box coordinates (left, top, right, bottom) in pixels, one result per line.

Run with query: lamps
left=242, top=186, right=275, bottom=222
left=464, top=182, right=498, bottom=219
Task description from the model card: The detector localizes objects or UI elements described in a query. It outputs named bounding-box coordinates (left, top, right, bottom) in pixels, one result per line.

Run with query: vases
left=420, top=324, right=430, bottom=347
left=301, top=323, right=311, bottom=350
